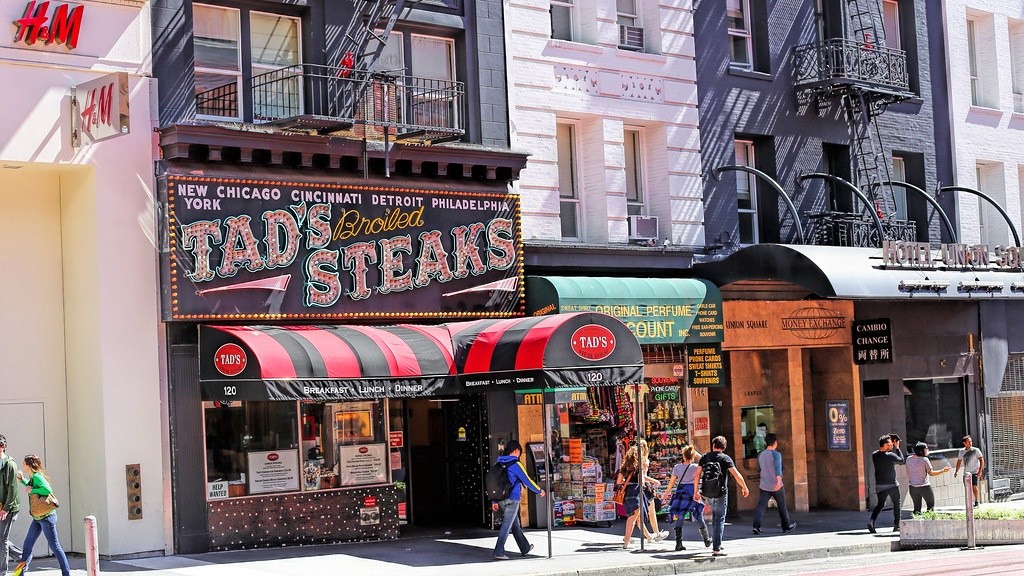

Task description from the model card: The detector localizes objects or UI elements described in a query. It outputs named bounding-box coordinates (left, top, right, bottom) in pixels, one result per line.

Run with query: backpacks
left=486, top=459, right=526, bottom=502
left=702, top=455, right=725, bottom=498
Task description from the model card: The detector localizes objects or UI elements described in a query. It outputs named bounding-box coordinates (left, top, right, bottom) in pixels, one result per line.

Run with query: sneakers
left=752, top=527, right=762, bottom=535
left=784, top=522, right=796, bottom=533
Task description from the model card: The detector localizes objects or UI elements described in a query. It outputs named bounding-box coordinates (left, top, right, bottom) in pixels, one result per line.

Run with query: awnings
left=193, top=323, right=461, bottom=402
left=525, top=272, right=728, bottom=346
left=708, top=152, right=1024, bottom=300
left=447, top=311, right=647, bottom=556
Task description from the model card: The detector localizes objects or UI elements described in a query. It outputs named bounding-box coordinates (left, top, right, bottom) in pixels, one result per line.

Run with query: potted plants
left=900, top=507, right=1024, bottom=546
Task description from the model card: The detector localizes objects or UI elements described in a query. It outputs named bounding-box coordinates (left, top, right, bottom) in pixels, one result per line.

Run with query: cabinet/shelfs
left=644, top=418, right=689, bottom=489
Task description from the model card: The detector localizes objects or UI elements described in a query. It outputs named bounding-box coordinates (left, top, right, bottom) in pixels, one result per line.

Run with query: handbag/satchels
left=612, top=489, right=625, bottom=506
left=667, top=493, right=675, bottom=506
left=889, top=434, right=901, bottom=442
left=30, top=494, right=59, bottom=520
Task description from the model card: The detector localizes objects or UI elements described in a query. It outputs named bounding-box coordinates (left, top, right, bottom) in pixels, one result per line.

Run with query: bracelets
left=941, top=469, right=944, bottom=472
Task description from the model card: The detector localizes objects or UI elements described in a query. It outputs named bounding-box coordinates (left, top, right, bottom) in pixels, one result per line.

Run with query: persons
left=954, top=436, right=984, bottom=507
left=693, top=436, right=750, bottom=556
left=866, top=435, right=904, bottom=533
left=906, top=442, right=951, bottom=515
left=662, top=445, right=713, bottom=551
left=490, top=440, right=546, bottom=559
left=752, top=433, right=797, bottom=534
left=0, top=434, right=34, bottom=576
left=616, top=439, right=669, bottom=550
left=17, top=454, right=71, bottom=576
left=753, top=422, right=767, bottom=453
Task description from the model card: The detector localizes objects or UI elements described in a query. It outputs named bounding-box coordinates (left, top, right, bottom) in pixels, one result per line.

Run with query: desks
left=553, top=481, right=616, bottom=528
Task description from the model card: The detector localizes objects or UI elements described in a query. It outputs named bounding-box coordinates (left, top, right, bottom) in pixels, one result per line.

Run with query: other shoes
left=893, top=525, right=900, bottom=532
left=623, top=543, right=635, bottom=549
left=867, top=520, right=877, bottom=534
left=494, top=555, right=509, bottom=559
left=23, top=554, right=33, bottom=572
left=623, top=537, right=637, bottom=544
left=523, top=544, right=535, bottom=558
left=654, top=529, right=670, bottom=543
left=647, top=533, right=655, bottom=543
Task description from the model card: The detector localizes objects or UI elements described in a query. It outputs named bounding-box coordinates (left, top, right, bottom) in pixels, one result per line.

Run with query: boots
left=699, top=526, right=713, bottom=547
left=673, top=526, right=687, bottom=551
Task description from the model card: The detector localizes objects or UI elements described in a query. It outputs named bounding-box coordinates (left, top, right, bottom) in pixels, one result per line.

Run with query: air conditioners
left=627, top=215, right=660, bottom=241
left=617, top=24, right=645, bottom=51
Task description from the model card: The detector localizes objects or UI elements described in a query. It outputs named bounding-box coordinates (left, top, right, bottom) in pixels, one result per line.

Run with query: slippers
left=712, top=551, right=727, bottom=556
left=720, top=546, right=724, bottom=550
